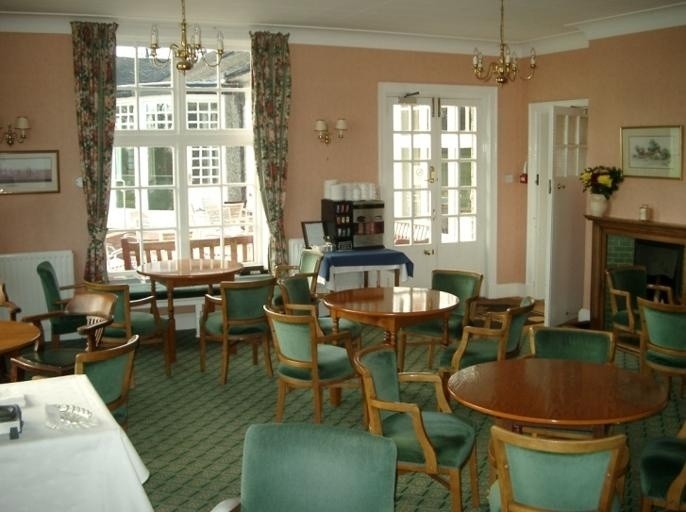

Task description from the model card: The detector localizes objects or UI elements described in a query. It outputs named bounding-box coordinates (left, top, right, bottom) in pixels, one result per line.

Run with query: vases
left=589, top=193, right=608, bottom=218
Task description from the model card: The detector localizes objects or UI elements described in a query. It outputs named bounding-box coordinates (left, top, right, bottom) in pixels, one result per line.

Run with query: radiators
left=288, top=238, right=364, bottom=317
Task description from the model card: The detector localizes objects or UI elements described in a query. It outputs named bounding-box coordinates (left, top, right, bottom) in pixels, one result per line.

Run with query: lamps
left=149, top=0, right=224, bottom=75
left=472, top=0, right=536, bottom=88
left=312, top=118, right=348, bottom=145
left=0, top=116, right=32, bottom=145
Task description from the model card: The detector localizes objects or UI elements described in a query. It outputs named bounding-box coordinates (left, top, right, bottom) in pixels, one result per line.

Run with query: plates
left=54, top=404, right=93, bottom=425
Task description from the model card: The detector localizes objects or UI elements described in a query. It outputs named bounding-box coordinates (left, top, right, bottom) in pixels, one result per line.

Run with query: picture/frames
left=0, top=150, right=60, bottom=195
left=620, top=125, right=683, bottom=180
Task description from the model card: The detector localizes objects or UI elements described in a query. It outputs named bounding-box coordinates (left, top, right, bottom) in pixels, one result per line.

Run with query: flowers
left=579, top=165, right=624, bottom=200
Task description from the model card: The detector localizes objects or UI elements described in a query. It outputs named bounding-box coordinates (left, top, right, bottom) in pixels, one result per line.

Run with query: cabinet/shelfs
left=320, top=198, right=385, bottom=250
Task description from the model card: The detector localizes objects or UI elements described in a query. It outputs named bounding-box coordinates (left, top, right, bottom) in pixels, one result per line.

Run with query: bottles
left=639, top=204, right=651, bottom=225
